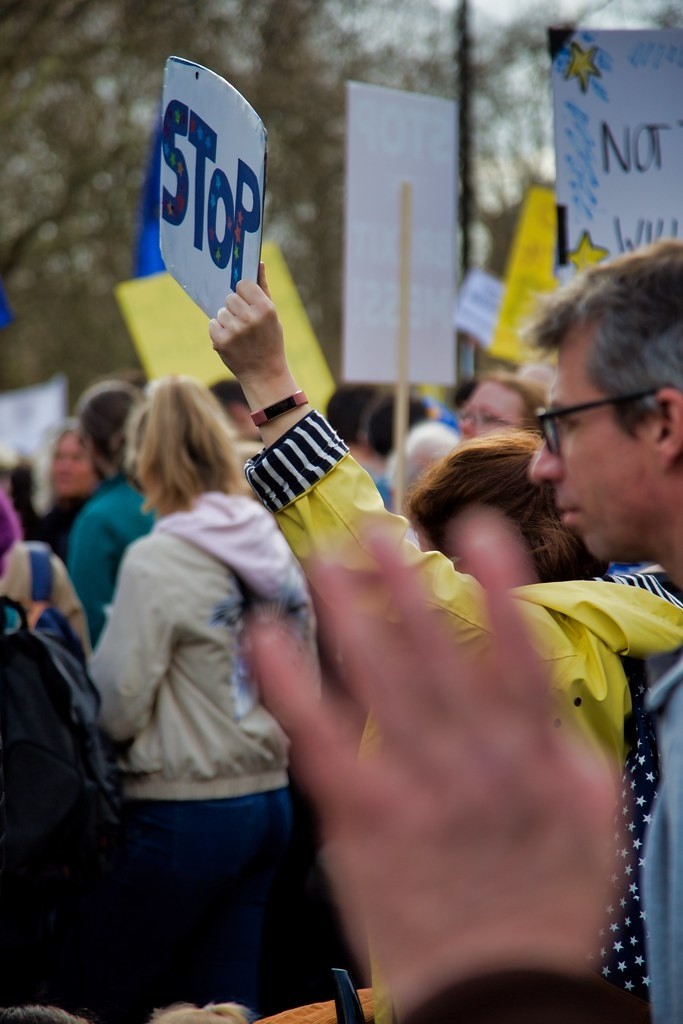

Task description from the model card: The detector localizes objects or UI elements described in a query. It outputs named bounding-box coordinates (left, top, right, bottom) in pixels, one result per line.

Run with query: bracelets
left=250, top=389, right=308, bottom=427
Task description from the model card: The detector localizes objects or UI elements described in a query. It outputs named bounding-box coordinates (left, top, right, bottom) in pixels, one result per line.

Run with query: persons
left=0, top=241, right=683, bottom=1024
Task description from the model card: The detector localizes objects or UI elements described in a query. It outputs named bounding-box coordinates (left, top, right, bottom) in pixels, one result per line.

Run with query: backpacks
left=0, top=553, right=103, bottom=872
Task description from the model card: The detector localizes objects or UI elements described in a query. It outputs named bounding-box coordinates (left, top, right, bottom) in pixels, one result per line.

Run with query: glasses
left=536, top=385, right=683, bottom=456
